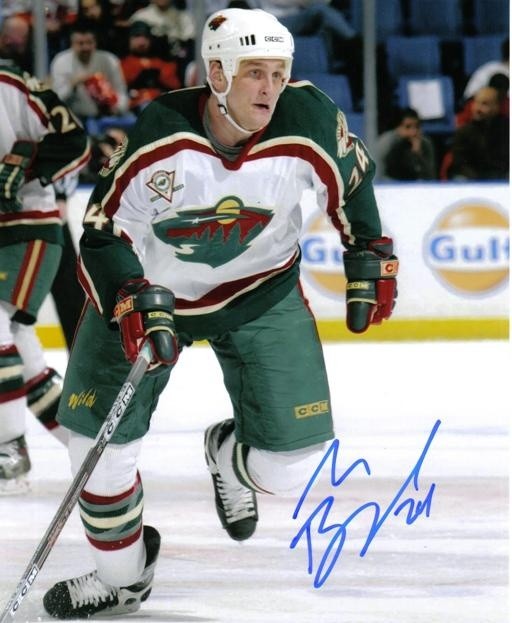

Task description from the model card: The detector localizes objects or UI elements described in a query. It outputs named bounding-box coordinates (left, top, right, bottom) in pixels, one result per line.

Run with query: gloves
left=0, top=139, right=39, bottom=211
left=113, top=279, right=178, bottom=372
left=342, top=237, right=399, bottom=333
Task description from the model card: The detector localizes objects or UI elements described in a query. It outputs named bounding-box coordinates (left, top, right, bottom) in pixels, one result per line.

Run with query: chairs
left=284, top=1, right=508, bottom=149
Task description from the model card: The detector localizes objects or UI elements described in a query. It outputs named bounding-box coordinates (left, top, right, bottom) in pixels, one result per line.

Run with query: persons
left=42, top=7, right=401, bottom=620
left=0, top=62, right=95, bottom=480
left=1, top=1, right=508, bottom=353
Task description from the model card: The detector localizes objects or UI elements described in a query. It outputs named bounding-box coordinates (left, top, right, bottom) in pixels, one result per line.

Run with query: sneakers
left=0, top=437, right=30, bottom=477
left=204, top=418, right=258, bottom=541
left=43, top=525, right=160, bottom=618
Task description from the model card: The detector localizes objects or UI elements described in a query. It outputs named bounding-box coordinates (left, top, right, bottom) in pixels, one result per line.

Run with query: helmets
left=201, top=8, right=295, bottom=96
